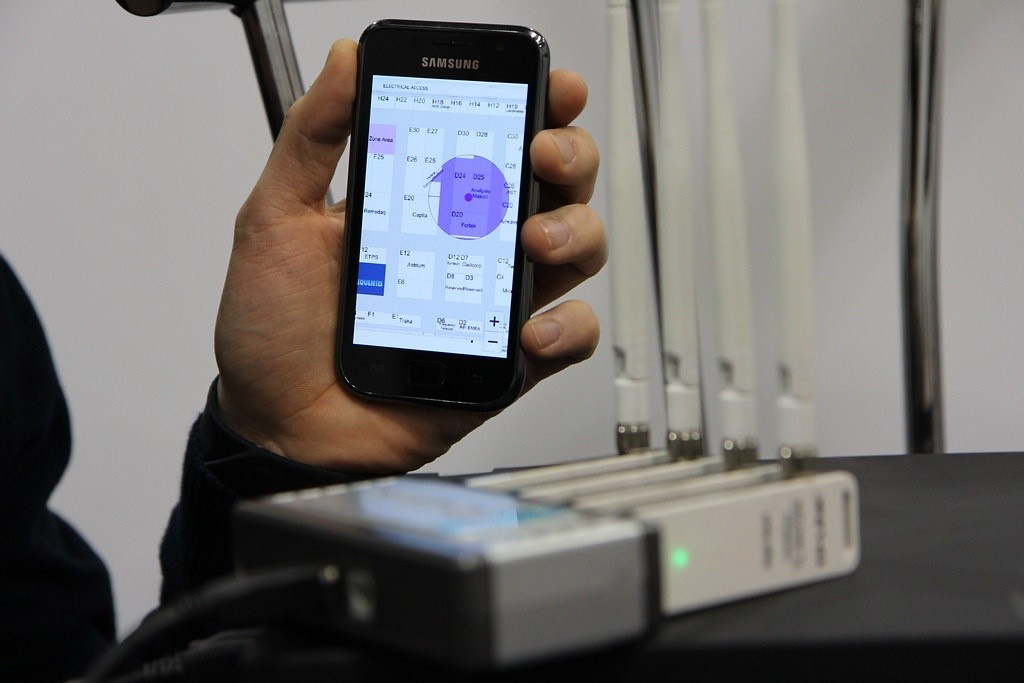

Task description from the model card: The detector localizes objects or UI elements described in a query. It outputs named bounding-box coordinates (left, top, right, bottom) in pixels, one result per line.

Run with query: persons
left=1, top=37, right=607, bottom=683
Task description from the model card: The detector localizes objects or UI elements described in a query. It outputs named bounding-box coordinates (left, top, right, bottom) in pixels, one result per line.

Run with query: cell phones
left=338, top=18, right=548, bottom=412
